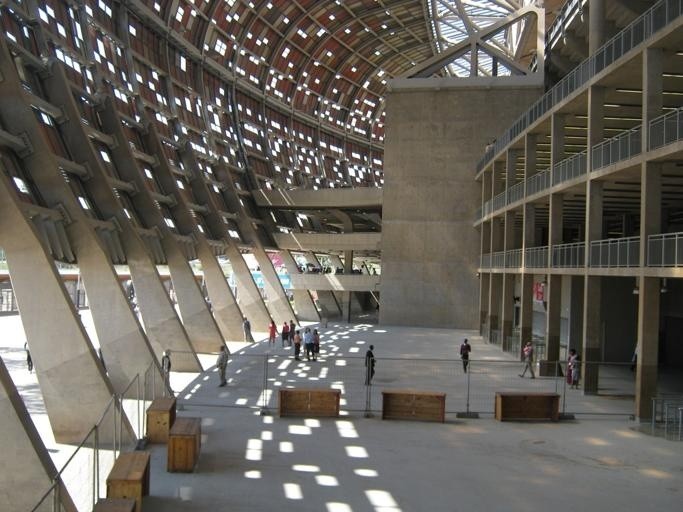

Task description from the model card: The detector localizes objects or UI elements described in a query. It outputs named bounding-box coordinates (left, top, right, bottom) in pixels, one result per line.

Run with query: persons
left=296, top=262, right=376, bottom=275
left=460, top=337, right=470, bottom=373
left=215, top=346, right=228, bottom=387
left=629, top=342, right=636, bottom=378
left=567, top=348, right=580, bottom=390
left=24, top=342, right=33, bottom=374
left=518, top=341, right=534, bottom=379
left=243, top=317, right=251, bottom=342
left=160, top=349, right=171, bottom=386
left=363, top=344, right=376, bottom=385
left=268, top=319, right=319, bottom=361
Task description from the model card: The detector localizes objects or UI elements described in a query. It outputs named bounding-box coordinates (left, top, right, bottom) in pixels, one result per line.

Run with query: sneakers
left=569, top=386, right=580, bottom=390
left=463, top=366, right=467, bottom=373
left=518, top=374, right=536, bottom=380
left=218, top=381, right=227, bottom=387
left=365, top=382, right=372, bottom=386
left=294, top=356, right=318, bottom=362
